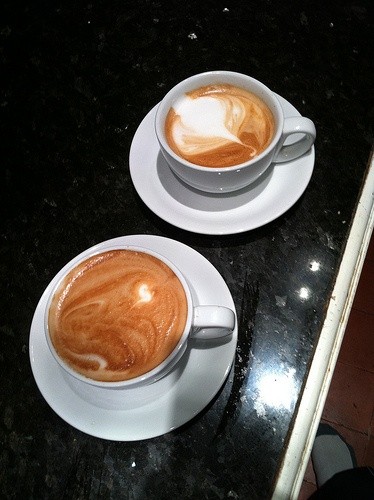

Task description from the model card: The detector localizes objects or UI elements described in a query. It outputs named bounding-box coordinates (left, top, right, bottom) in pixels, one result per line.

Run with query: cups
left=43, top=245, right=236, bottom=392
left=154, top=71, right=317, bottom=193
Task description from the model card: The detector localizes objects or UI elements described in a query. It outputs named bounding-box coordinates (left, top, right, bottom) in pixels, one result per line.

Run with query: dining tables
left=0, top=1, right=374, bottom=499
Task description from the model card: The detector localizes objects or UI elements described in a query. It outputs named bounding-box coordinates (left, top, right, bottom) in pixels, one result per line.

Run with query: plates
left=129, top=91, right=316, bottom=236
left=29, top=234, right=239, bottom=442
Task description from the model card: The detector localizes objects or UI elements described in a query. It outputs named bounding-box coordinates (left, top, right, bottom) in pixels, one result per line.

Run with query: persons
left=309, top=423, right=374, bottom=500
